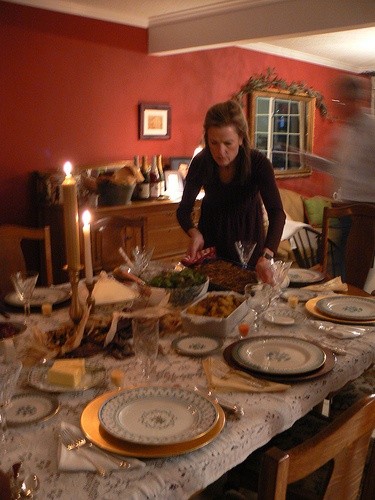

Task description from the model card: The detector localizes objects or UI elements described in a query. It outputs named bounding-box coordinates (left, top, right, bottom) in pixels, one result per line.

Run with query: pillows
left=302, top=197, right=332, bottom=228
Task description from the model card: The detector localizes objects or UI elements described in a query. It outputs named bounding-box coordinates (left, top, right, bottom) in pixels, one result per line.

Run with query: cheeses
left=47, top=358, right=85, bottom=388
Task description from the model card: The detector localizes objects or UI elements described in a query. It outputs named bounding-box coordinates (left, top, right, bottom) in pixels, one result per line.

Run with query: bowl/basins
left=179, top=292, right=248, bottom=337
left=137, top=269, right=209, bottom=312
left=96, top=181, right=136, bottom=206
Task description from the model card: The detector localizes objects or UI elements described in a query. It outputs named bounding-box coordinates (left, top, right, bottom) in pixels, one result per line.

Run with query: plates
left=0, top=321, right=27, bottom=344
left=171, top=334, right=223, bottom=356
left=2, top=286, right=72, bottom=307
left=222, top=268, right=375, bottom=382
left=27, top=359, right=105, bottom=393
left=4, top=393, right=60, bottom=428
left=80, top=384, right=226, bottom=458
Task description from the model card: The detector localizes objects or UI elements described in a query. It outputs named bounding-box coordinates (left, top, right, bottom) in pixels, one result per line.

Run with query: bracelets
left=260, top=252, right=273, bottom=260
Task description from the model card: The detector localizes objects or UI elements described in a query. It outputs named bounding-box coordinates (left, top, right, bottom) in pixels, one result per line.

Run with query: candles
left=61, top=160, right=80, bottom=272
left=80, top=209, right=94, bottom=285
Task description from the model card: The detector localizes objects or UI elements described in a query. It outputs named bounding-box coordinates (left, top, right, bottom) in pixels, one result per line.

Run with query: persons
left=177, top=100, right=286, bottom=285
left=298, top=75, right=375, bottom=288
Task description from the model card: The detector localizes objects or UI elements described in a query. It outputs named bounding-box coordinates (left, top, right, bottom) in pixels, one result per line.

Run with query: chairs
left=318, top=203, right=375, bottom=295
left=288, top=226, right=346, bottom=283
left=0, top=224, right=53, bottom=304
left=223, top=392, right=375, bottom=500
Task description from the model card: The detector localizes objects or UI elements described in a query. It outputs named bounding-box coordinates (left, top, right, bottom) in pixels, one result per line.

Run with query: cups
left=131, top=243, right=154, bottom=274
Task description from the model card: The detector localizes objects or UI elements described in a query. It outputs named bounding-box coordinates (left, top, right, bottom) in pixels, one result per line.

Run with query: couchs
left=260, top=187, right=343, bottom=270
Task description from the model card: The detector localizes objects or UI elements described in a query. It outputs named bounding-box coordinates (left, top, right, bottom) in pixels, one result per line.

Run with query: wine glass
left=235, top=241, right=256, bottom=277
left=267, top=257, right=293, bottom=306
left=12, top=271, right=40, bottom=326
left=131, top=317, right=160, bottom=383
left=244, top=284, right=272, bottom=336
left=0, top=359, right=24, bottom=454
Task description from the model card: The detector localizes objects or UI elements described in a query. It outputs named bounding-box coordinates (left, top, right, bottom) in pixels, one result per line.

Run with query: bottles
left=133, top=153, right=167, bottom=200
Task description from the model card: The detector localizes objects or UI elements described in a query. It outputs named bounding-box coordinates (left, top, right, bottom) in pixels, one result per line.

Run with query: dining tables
left=0, top=275, right=375, bottom=500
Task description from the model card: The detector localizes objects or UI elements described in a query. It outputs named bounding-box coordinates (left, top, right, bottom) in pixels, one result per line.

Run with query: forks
left=56, top=426, right=131, bottom=479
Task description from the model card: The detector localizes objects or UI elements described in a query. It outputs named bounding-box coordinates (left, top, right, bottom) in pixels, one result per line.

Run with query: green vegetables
left=143, top=267, right=208, bottom=304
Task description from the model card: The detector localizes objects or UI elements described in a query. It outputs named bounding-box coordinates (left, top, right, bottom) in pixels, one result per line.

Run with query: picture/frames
left=138, top=102, right=171, bottom=140
left=164, top=156, right=191, bottom=201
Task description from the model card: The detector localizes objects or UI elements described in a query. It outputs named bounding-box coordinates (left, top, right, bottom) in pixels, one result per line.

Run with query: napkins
left=299, top=275, right=349, bottom=293
left=56, top=422, right=146, bottom=473
left=202, top=353, right=290, bottom=395
left=310, top=320, right=369, bottom=339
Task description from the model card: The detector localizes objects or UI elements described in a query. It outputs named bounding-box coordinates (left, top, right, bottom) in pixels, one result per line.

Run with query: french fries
left=188, top=295, right=239, bottom=319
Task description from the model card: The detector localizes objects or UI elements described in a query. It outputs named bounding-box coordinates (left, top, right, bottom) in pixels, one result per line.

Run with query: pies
left=199, top=260, right=257, bottom=291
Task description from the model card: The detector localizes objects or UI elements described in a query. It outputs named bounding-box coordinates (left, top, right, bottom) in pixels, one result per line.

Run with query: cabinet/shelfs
left=39, top=189, right=206, bottom=287
